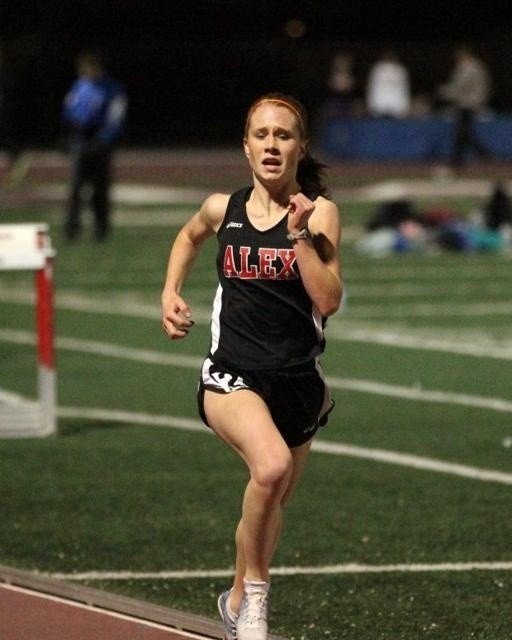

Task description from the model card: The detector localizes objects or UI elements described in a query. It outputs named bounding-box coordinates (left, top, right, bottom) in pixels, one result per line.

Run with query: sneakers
left=235, top=576, right=272, bottom=640
left=217, top=589, right=240, bottom=640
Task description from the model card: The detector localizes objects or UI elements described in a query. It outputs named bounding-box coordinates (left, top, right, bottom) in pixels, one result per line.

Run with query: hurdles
left=0, top=222, right=57, bottom=442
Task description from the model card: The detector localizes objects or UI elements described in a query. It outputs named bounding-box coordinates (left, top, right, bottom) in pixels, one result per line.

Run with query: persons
left=362, top=33, right=512, bottom=177
left=161, top=91, right=344, bottom=640
left=61, top=36, right=131, bottom=243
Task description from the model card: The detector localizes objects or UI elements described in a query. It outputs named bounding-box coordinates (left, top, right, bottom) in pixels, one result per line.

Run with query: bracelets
left=286, top=227, right=310, bottom=241
